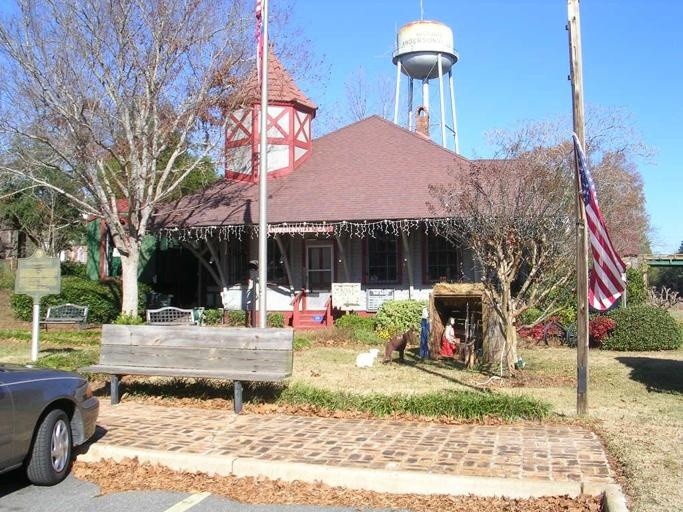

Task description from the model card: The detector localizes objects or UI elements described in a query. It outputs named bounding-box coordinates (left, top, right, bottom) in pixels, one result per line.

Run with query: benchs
left=79, top=306, right=296, bottom=413
left=38, top=302, right=90, bottom=332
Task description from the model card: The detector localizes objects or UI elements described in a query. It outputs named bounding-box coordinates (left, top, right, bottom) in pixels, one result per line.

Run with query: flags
left=253, top=0, right=265, bottom=87
left=572, top=135, right=628, bottom=314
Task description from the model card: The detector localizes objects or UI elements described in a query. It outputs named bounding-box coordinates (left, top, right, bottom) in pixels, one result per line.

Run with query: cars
left=1, top=363, right=100, bottom=486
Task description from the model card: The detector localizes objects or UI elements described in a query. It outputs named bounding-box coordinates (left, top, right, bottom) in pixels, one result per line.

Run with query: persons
left=439, top=316, right=459, bottom=357
